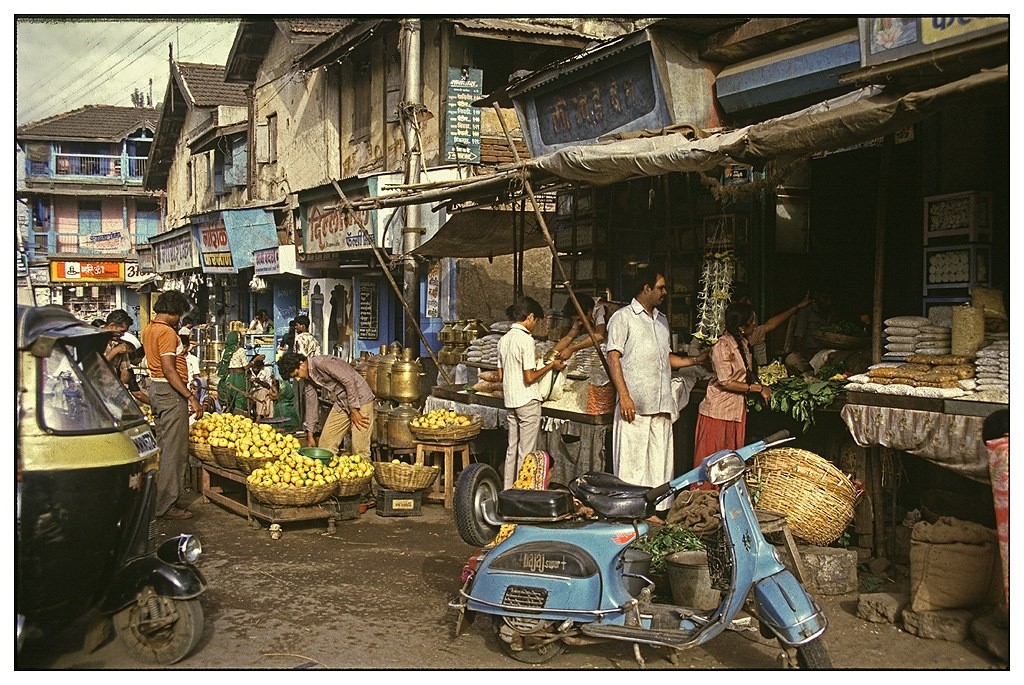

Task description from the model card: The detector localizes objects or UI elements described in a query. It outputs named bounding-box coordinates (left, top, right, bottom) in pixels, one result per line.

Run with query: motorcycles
left=16, top=302, right=207, bottom=669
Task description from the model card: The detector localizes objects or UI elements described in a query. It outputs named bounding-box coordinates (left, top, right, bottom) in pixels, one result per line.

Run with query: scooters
left=446, top=430, right=833, bottom=670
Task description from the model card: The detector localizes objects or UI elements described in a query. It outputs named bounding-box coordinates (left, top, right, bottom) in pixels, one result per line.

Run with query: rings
left=625, top=411, right=627, bottom=415
left=632, top=410, right=634, bottom=412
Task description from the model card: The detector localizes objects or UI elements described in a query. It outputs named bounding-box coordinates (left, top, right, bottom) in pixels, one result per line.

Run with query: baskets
left=187, top=441, right=219, bottom=462
left=333, top=463, right=375, bottom=496
left=409, top=410, right=482, bottom=442
left=245, top=468, right=338, bottom=506
left=748, top=445, right=865, bottom=544
left=371, top=461, right=443, bottom=493
left=234, top=451, right=280, bottom=475
left=210, top=445, right=240, bottom=469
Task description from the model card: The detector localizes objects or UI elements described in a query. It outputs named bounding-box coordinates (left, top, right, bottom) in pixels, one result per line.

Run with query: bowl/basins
left=299, top=446, right=334, bottom=466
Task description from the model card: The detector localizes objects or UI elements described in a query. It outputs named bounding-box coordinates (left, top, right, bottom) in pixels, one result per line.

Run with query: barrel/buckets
left=665, top=550, right=722, bottom=610
left=622, top=549, right=652, bottom=598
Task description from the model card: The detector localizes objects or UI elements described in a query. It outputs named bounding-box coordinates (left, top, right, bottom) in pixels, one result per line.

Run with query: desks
left=199, top=460, right=254, bottom=525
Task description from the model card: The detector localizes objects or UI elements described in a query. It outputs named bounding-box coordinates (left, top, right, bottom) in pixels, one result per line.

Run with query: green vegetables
left=817, top=319, right=872, bottom=338
left=746, top=361, right=853, bottom=433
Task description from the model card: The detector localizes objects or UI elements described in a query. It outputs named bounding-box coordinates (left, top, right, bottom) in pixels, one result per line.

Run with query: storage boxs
left=376, top=489, right=424, bottom=517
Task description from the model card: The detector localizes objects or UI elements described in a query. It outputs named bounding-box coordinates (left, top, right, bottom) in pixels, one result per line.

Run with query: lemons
left=189, top=412, right=373, bottom=487
left=412, top=409, right=471, bottom=429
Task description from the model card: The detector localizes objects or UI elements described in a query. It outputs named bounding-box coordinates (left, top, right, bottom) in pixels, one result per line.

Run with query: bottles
left=350, top=344, right=426, bottom=448
left=438, top=318, right=481, bottom=364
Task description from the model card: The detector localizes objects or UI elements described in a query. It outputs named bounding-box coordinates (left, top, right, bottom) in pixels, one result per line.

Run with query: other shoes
left=156, top=505, right=193, bottom=521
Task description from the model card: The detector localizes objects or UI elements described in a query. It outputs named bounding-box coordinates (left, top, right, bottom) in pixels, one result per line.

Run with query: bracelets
left=694, top=357, right=697, bottom=364
left=187, top=393, right=192, bottom=399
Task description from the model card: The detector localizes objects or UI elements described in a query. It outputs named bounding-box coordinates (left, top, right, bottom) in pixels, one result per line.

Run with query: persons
left=269, top=379, right=300, bottom=431
left=606, top=269, right=709, bottom=524
left=249, top=309, right=273, bottom=332
left=487, top=450, right=555, bottom=546
left=689, top=290, right=811, bottom=490
left=55, top=309, right=151, bottom=408
left=293, top=315, right=321, bottom=430
left=142, top=289, right=203, bottom=519
left=498, top=297, right=565, bottom=490
left=552, top=292, right=612, bottom=359
left=277, top=352, right=376, bottom=462
left=216, top=331, right=266, bottom=410
left=248, top=354, right=273, bottom=419
left=279, top=321, right=295, bottom=352
left=794, top=284, right=840, bottom=355
left=187, top=378, right=222, bottom=414
left=179, top=334, right=200, bottom=391
left=178, top=316, right=198, bottom=347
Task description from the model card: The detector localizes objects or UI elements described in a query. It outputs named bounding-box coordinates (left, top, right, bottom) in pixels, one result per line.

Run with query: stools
left=374, top=442, right=417, bottom=465
left=411, top=439, right=469, bottom=509
left=711, top=508, right=818, bottom=602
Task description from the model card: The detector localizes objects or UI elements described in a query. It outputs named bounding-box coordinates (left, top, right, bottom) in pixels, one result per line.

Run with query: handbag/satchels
left=535, top=358, right=568, bottom=401
